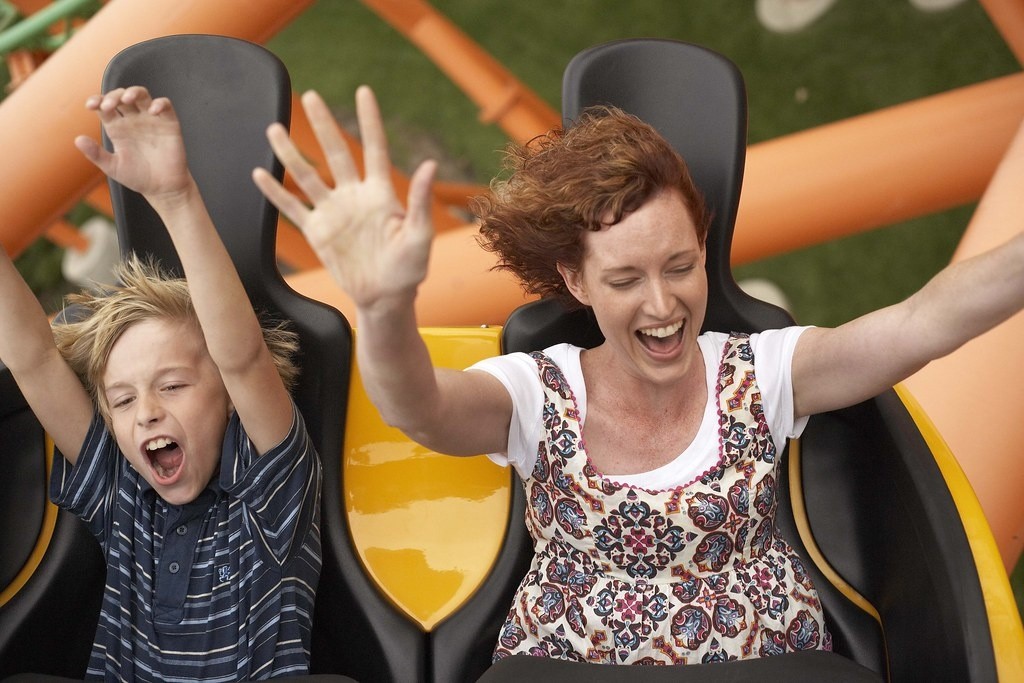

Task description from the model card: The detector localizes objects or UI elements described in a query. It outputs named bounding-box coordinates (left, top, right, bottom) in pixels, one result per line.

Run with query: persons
left=250, top=89, right=1024, bottom=660
left=0, top=87, right=324, bottom=681
